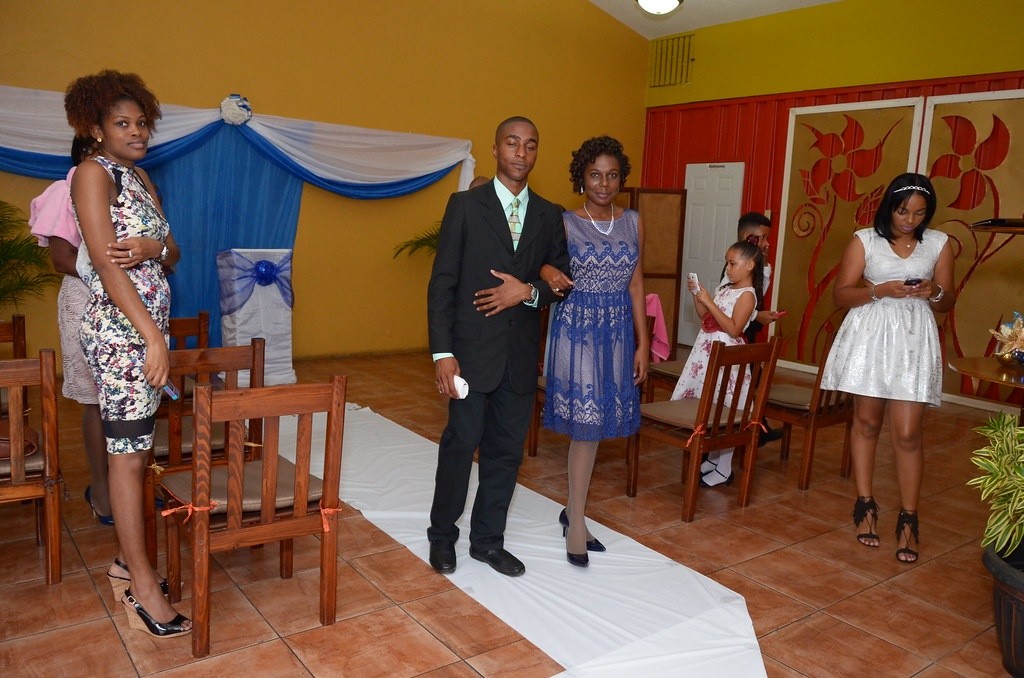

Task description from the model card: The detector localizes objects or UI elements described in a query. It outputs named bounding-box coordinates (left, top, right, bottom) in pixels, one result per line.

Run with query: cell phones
left=689, top=273, right=700, bottom=296
left=163, top=380, right=181, bottom=401
left=904, top=278, right=923, bottom=286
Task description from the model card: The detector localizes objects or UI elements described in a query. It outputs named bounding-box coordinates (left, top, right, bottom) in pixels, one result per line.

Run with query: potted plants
left=964, top=406, right=1024, bottom=678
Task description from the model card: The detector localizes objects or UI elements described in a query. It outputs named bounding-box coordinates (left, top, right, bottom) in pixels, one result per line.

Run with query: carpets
left=235, top=387, right=767, bottom=678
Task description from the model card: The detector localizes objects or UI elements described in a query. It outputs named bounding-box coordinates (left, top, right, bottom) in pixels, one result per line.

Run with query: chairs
left=159, top=374, right=352, bottom=659
left=756, top=327, right=855, bottom=491
left=526, top=295, right=659, bottom=465
left=143, top=336, right=266, bottom=570
left=625, top=336, right=782, bottom=523
left=0, top=349, right=63, bottom=584
left=158, top=310, right=210, bottom=401
left=644, top=360, right=689, bottom=406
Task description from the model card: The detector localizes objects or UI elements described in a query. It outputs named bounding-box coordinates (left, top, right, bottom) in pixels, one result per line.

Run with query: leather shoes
left=469, top=545, right=526, bottom=577
left=429, top=539, right=457, bottom=573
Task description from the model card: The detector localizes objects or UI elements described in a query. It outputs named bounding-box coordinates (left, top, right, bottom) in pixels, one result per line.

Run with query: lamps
left=635, top=0, right=684, bottom=16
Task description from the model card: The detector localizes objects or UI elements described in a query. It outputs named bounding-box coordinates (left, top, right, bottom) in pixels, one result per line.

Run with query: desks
left=948, top=357, right=1024, bottom=427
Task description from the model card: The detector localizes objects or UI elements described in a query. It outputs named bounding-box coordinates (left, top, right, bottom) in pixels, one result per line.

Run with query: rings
left=555, top=288, right=559, bottom=292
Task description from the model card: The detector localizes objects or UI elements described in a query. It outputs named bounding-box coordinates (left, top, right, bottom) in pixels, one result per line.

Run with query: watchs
left=154, top=242, right=170, bottom=264
left=523, top=282, right=537, bottom=304
left=928, top=284, right=944, bottom=302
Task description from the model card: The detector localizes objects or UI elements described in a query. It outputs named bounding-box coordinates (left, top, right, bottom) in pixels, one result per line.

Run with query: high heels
left=107, top=558, right=178, bottom=602
left=154, top=497, right=165, bottom=509
left=565, top=527, right=589, bottom=568
left=84, top=484, right=116, bottom=525
left=896, top=509, right=919, bottom=563
left=852, top=496, right=881, bottom=547
left=698, top=469, right=735, bottom=487
left=559, top=508, right=606, bottom=552
left=120, top=588, right=194, bottom=639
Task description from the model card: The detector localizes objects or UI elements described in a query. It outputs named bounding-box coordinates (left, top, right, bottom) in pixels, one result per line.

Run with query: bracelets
left=869, top=285, right=881, bottom=302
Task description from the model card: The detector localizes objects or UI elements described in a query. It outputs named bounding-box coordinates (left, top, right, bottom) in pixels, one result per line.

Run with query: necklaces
left=891, top=230, right=919, bottom=249
left=583, top=202, right=616, bottom=236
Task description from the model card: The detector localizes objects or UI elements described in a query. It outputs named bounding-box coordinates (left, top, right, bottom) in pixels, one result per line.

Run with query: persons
left=29, top=130, right=168, bottom=528
left=820, top=174, right=958, bottom=563
left=669, top=234, right=765, bottom=487
left=426, top=116, right=573, bottom=578
left=720, top=211, right=787, bottom=449
left=539, top=135, right=651, bottom=567
left=65, top=70, right=196, bottom=640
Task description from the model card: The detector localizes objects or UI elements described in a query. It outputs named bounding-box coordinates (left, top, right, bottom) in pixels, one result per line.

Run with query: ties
left=508, top=198, right=522, bottom=252
